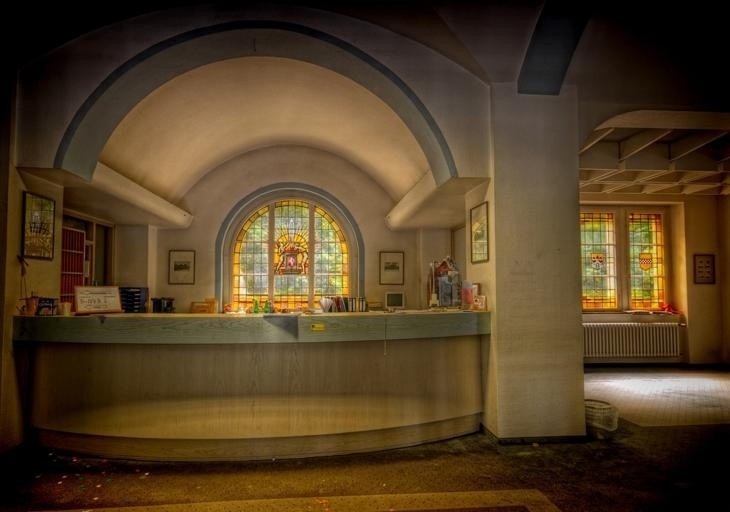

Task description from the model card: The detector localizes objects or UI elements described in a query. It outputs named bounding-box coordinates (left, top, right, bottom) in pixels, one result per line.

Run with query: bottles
left=264, top=301, right=269, bottom=314
left=254, top=301, right=258, bottom=313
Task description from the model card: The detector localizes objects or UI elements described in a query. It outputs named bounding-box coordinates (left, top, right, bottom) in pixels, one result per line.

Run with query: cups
left=62, top=302, right=72, bottom=315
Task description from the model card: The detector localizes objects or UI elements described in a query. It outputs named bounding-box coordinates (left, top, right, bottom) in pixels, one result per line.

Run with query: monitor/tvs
left=385, top=292, right=408, bottom=309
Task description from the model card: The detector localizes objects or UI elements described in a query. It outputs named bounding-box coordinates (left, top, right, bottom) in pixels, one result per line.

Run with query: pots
left=150, top=297, right=176, bottom=313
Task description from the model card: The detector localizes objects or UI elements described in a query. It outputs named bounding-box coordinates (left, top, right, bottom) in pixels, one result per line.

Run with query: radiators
left=583, top=323, right=687, bottom=358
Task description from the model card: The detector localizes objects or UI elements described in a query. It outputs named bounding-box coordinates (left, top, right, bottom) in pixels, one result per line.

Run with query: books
left=319, top=297, right=368, bottom=312
left=61, top=229, right=93, bottom=314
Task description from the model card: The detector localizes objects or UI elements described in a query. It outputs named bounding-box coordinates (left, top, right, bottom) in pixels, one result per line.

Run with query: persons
left=276, top=242, right=289, bottom=271
left=294, top=241, right=308, bottom=275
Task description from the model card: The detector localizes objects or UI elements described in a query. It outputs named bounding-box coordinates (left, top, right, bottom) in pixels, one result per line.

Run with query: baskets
left=585, top=396, right=621, bottom=441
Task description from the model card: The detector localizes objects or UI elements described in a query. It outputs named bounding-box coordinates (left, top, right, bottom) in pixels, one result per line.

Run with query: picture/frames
left=20, top=191, right=56, bottom=260
left=693, top=254, right=716, bottom=284
left=168, top=249, right=195, bottom=285
left=470, top=201, right=489, bottom=263
left=379, top=251, right=404, bottom=285
